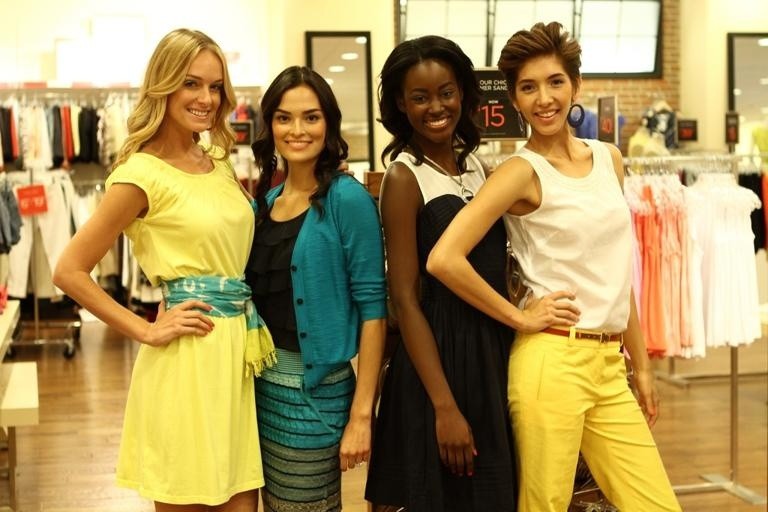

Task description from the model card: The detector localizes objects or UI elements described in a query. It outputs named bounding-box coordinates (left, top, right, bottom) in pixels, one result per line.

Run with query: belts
left=543, top=327, right=623, bottom=342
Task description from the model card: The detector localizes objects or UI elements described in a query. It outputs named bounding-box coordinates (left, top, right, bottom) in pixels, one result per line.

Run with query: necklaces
left=425, top=149, right=475, bottom=203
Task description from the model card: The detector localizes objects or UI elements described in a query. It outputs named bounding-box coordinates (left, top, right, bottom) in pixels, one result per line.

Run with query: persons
left=361, top=34, right=518, bottom=512
left=424, top=17, right=683, bottom=512
left=52, top=28, right=277, bottom=512
left=242, top=66, right=389, bottom=512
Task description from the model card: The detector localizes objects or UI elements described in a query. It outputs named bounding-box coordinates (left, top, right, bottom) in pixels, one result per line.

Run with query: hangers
left=621, top=153, right=739, bottom=203
left=0, top=86, right=135, bottom=116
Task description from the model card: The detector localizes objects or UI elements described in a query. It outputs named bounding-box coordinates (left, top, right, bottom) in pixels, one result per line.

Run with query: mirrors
left=725, top=32, right=766, bottom=174
left=304, top=31, right=374, bottom=186
